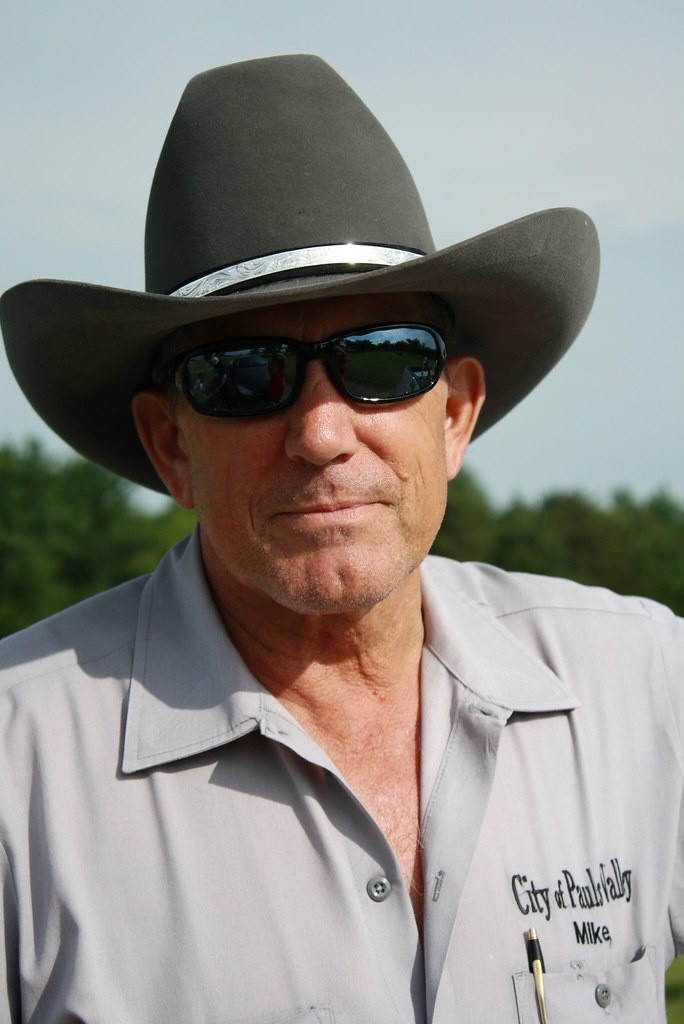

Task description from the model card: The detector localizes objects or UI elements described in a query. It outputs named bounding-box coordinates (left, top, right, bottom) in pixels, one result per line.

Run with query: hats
left=0, top=53, right=601, bottom=496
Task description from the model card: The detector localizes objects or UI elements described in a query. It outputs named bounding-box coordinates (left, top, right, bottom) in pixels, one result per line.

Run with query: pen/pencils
left=526, top=927, right=549, bottom=1024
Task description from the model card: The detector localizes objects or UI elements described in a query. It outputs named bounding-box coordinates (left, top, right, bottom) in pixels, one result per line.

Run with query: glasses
left=156, top=323, right=448, bottom=420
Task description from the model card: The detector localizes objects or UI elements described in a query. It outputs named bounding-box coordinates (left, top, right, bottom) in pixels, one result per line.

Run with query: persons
left=0, top=52, right=682, bottom=1024
